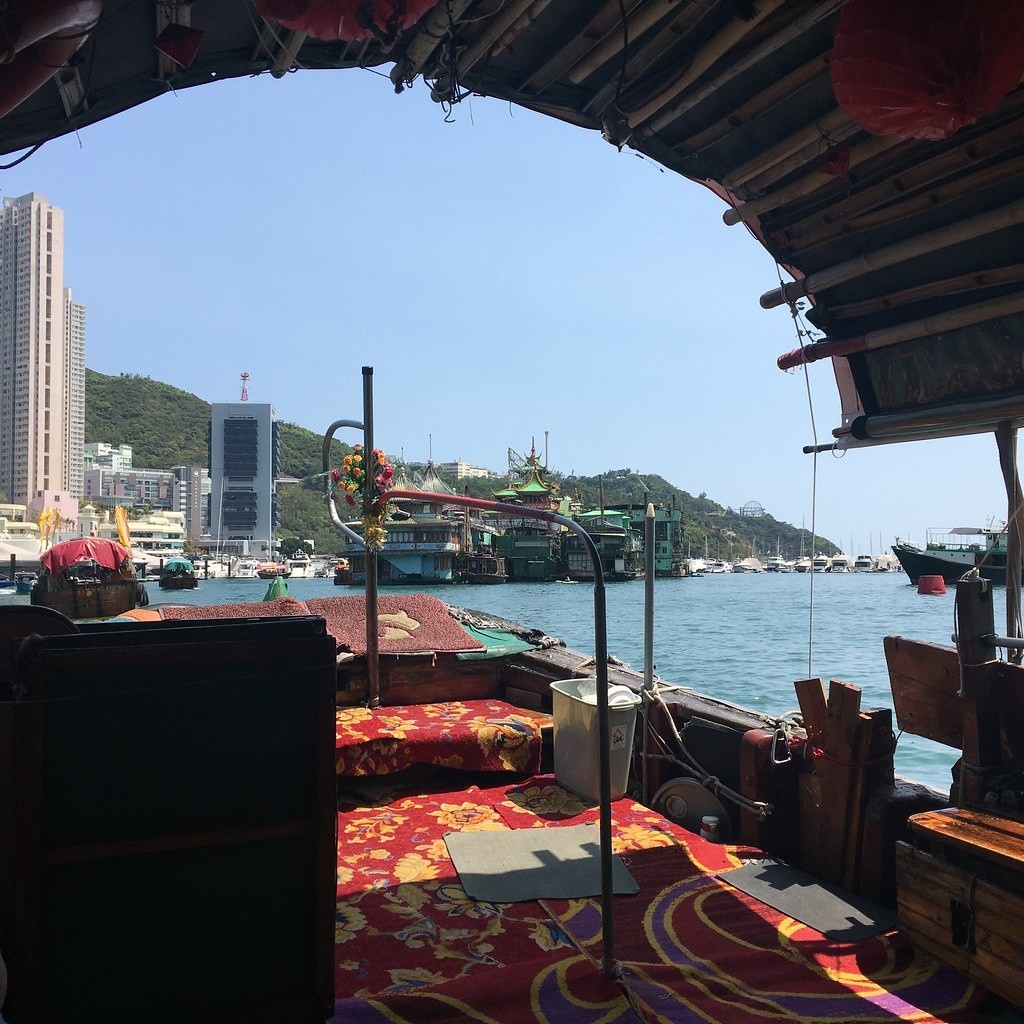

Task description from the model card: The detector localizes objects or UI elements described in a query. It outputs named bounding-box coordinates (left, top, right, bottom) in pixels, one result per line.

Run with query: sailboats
left=685, top=514, right=905, bottom=573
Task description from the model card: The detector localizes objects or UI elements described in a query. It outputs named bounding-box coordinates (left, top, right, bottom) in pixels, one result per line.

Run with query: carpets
left=302, top=593, right=487, bottom=655
left=158, top=597, right=309, bottom=622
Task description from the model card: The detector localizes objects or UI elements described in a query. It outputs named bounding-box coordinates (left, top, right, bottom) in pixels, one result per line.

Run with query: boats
left=331, top=431, right=687, bottom=587
left=891, top=513, right=1024, bottom=585
left=29, top=537, right=149, bottom=620
left=159, top=558, right=199, bottom=590
left=563, top=581, right=579, bottom=583
left=13, top=571, right=38, bottom=595
left=690, top=574, right=704, bottom=577
left=0, top=574, right=15, bottom=588
left=182, top=551, right=259, bottom=577
left=256, top=548, right=316, bottom=579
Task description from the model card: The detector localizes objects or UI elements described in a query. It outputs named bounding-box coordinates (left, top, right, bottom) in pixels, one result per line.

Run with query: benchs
left=334, top=699, right=553, bottom=784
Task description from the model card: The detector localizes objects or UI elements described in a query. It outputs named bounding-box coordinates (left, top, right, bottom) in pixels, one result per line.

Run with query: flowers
left=321, top=444, right=395, bottom=552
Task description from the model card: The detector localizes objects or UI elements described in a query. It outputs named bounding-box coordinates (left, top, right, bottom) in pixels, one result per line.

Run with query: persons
left=99, top=566, right=112, bottom=584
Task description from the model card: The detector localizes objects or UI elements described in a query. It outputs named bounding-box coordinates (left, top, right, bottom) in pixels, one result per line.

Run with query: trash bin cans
left=549, top=678, right=643, bottom=805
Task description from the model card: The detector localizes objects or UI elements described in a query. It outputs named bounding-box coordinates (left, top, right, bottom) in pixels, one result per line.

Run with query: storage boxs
left=896, top=807, right=1024, bottom=1010
